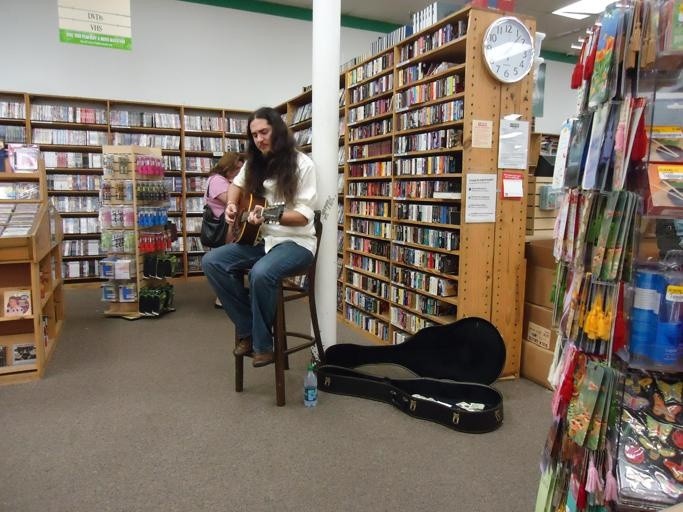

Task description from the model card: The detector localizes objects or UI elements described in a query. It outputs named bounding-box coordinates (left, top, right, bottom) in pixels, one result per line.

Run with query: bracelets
left=227, top=201, right=239, bottom=205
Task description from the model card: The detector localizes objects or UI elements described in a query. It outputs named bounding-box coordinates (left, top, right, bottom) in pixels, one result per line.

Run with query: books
left=0, top=345, right=7, bottom=367
left=341, top=2, right=469, bottom=346
left=287, top=275, right=307, bottom=288
left=281, top=103, right=313, bottom=161
left=1, top=94, right=248, bottom=278
left=12, top=343, right=36, bottom=365
left=3, top=290, right=32, bottom=317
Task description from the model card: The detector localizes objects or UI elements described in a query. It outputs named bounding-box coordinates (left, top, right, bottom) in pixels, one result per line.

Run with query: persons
left=200, top=107, right=318, bottom=367
left=204, top=150, right=245, bottom=309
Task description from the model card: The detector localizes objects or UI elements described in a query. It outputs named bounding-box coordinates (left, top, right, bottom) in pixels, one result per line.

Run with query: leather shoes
left=252, top=349, right=273, bottom=367
left=233, top=338, right=252, bottom=355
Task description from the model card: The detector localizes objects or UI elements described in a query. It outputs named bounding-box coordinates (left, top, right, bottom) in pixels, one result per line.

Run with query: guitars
left=225, top=193, right=284, bottom=244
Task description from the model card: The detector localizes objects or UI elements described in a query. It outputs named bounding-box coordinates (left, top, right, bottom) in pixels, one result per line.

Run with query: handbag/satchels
left=200, top=204, right=228, bottom=248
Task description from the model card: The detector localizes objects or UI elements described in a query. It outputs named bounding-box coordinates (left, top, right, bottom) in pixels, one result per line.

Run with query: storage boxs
left=521, top=239, right=556, bottom=393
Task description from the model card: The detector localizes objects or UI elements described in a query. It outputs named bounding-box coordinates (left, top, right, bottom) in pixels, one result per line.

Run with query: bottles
left=303, top=367, right=317, bottom=406
